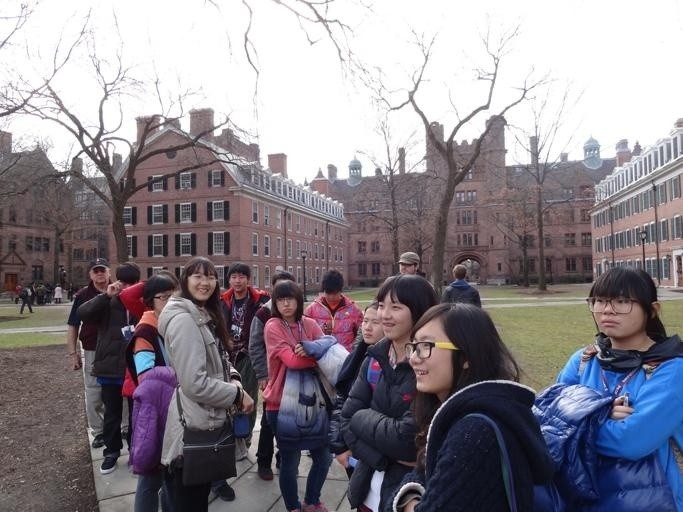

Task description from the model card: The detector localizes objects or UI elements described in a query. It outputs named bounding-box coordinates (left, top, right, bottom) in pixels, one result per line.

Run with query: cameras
left=232, top=333, right=242, bottom=342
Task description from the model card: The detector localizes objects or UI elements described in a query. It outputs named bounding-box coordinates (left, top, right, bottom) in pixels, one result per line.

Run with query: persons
left=66, top=256, right=364, bottom=511
left=334, top=252, right=555, bottom=511
left=556, top=265, right=683, bottom=512
left=13, top=281, right=74, bottom=314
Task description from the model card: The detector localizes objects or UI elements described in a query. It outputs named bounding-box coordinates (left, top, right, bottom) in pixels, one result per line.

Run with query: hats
left=396, top=251, right=422, bottom=266
left=86, top=257, right=111, bottom=273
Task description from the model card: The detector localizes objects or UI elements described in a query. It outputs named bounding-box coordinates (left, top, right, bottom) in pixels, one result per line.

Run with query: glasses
left=404, top=340, right=461, bottom=362
left=153, top=293, right=172, bottom=301
left=585, top=295, right=642, bottom=315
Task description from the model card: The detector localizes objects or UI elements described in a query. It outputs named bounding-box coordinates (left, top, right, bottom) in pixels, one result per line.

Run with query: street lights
left=650, top=178, right=661, bottom=286
left=325, top=219, right=331, bottom=272
left=283, top=205, right=289, bottom=272
left=606, top=201, right=616, bottom=266
left=637, top=229, right=647, bottom=271
left=299, top=249, right=309, bottom=302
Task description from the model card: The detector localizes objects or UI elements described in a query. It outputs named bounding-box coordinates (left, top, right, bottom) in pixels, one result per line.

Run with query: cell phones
left=73, top=363, right=82, bottom=370
left=623, top=392, right=629, bottom=407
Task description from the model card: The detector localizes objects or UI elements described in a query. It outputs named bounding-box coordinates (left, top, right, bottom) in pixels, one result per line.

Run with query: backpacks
left=20, top=288, right=29, bottom=300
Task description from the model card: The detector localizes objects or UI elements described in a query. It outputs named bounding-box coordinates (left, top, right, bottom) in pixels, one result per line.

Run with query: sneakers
left=300, top=499, right=330, bottom=512
left=214, top=482, right=236, bottom=502
left=290, top=507, right=301, bottom=512
left=99, top=455, right=119, bottom=475
left=235, top=448, right=248, bottom=462
left=91, top=434, right=107, bottom=449
left=121, top=431, right=129, bottom=440
left=257, top=460, right=274, bottom=480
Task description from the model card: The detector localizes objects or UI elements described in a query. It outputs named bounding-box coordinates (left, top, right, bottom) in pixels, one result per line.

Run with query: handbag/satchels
left=179, top=413, right=240, bottom=482
